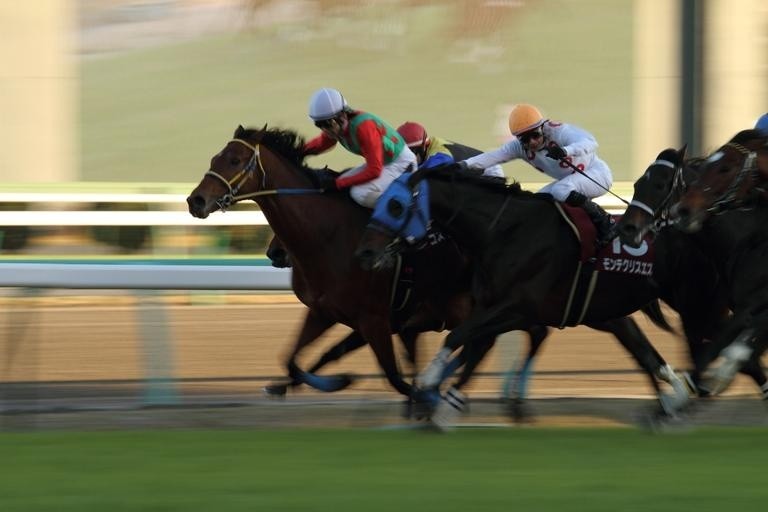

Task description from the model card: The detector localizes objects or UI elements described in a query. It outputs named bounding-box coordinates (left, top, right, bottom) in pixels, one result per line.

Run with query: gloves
left=546, top=147, right=566, bottom=160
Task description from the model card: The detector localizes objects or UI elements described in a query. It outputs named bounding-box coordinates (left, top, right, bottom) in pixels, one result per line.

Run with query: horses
left=185, top=122, right=545, bottom=422
left=344, top=159, right=689, bottom=424
left=615, top=126, right=768, bottom=428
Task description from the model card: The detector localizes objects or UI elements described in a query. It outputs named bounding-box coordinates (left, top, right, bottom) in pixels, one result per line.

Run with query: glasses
left=516, top=132, right=542, bottom=144
left=315, top=121, right=334, bottom=129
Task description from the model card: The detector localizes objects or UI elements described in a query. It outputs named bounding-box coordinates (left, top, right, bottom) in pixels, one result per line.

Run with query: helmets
left=509, top=104, right=545, bottom=136
left=308, top=87, right=348, bottom=121
left=396, top=121, right=430, bottom=148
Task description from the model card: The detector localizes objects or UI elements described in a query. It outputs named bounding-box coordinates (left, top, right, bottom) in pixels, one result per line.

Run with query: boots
left=582, top=197, right=618, bottom=251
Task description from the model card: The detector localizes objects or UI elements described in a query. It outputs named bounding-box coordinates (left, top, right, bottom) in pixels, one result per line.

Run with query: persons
left=292, top=90, right=418, bottom=213
left=451, top=104, right=618, bottom=243
left=395, top=121, right=506, bottom=187
